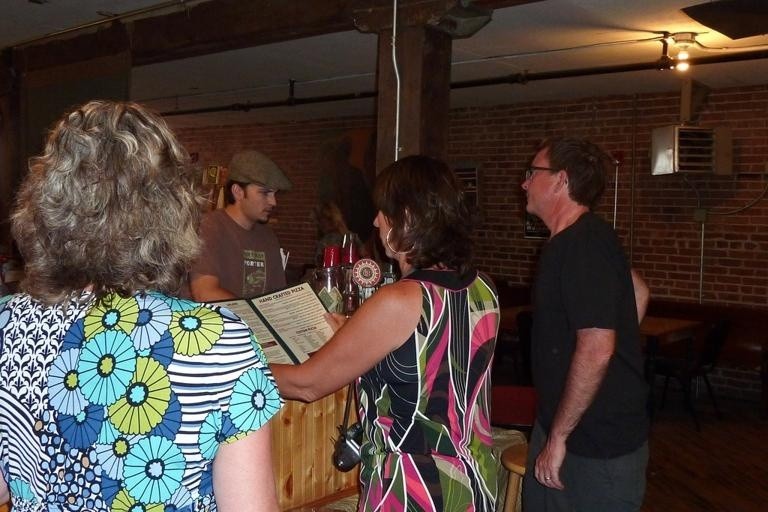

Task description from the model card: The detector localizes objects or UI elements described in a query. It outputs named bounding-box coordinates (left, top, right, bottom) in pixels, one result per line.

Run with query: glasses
left=525, top=166, right=568, bottom=185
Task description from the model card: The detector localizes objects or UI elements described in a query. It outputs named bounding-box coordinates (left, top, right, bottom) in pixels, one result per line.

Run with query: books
left=200, top=279, right=336, bottom=366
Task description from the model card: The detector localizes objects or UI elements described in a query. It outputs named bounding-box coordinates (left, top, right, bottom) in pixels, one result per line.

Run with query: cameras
left=330, top=420, right=362, bottom=474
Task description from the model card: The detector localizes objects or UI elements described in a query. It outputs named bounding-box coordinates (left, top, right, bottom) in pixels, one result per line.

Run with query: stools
left=503, top=444, right=531, bottom=511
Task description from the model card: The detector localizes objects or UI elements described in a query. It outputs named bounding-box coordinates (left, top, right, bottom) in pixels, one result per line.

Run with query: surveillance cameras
left=654, top=53, right=677, bottom=70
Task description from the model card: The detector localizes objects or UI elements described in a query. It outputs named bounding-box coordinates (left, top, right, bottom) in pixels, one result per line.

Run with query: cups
left=324, top=246, right=340, bottom=269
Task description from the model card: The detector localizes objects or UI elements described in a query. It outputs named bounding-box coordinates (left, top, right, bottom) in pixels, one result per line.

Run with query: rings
left=545, top=476, right=552, bottom=481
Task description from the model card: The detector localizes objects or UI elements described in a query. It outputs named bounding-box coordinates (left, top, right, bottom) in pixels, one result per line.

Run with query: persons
left=0, top=100, right=286, bottom=511
left=521, top=136, right=651, bottom=512
left=182, top=149, right=297, bottom=302
left=266, top=154, right=501, bottom=512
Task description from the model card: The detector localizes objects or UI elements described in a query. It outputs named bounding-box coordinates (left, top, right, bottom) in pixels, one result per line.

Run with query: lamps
left=674, top=33, right=693, bottom=73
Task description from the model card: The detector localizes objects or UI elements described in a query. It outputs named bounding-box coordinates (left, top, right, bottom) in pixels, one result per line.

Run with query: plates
left=353, top=259, right=381, bottom=288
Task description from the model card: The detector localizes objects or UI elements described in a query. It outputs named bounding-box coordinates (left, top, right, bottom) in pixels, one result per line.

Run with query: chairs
left=657, top=321, right=728, bottom=431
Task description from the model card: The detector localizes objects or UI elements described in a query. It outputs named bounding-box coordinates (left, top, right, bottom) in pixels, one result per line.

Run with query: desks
left=501, top=304, right=711, bottom=380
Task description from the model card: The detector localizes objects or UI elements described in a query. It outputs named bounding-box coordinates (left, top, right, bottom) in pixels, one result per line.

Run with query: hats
left=227, top=151, right=294, bottom=193
